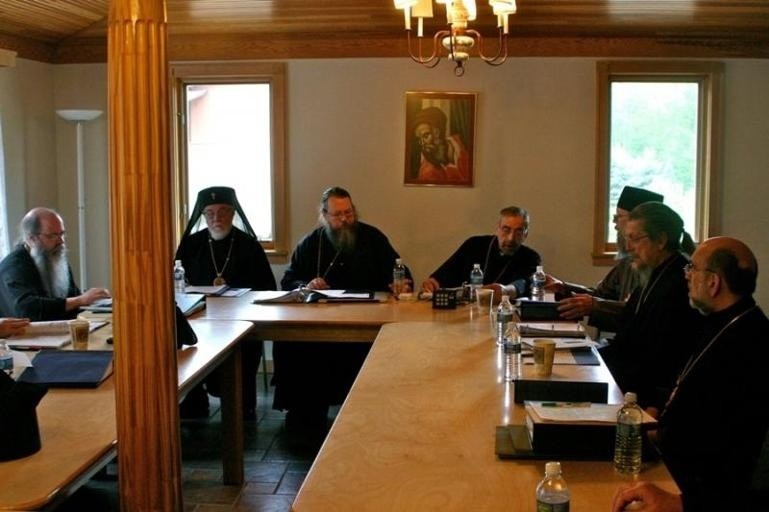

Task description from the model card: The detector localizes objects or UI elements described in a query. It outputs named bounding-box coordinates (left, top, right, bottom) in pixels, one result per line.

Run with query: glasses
left=27, top=230, right=65, bottom=241
left=610, top=212, right=627, bottom=222
left=622, top=232, right=649, bottom=245
left=201, top=205, right=235, bottom=219
left=325, top=208, right=351, bottom=219
left=683, top=263, right=716, bottom=273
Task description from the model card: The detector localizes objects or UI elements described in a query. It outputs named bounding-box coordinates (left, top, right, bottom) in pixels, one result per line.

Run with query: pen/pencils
left=542, top=401, right=592, bottom=407
left=10, top=346, right=41, bottom=351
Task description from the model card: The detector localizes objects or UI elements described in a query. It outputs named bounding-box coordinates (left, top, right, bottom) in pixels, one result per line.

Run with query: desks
left=1, top=291, right=254, bottom=508
left=292, top=316, right=682, bottom=512
left=75, top=290, right=566, bottom=341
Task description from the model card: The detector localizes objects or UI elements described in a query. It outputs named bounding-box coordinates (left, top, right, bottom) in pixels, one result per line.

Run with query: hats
left=616, top=185, right=665, bottom=215
left=199, top=189, right=232, bottom=212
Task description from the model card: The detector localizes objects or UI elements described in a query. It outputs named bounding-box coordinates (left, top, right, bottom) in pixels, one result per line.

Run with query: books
left=184, top=284, right=252, bottom=298
left=6, top=320, right=110, bottom=350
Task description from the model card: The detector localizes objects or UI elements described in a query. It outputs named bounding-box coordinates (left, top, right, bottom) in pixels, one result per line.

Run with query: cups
left=69, top=321, right=88, bottom=349
left=533, top=338, right=555, bottom=376
left=477, top=289, right=494, bottom=313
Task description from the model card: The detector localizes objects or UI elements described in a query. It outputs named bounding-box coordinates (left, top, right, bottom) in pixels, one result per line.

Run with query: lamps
left=394, top=0, right=517, bottom=77
left=55, top=109, right=103, bottom=294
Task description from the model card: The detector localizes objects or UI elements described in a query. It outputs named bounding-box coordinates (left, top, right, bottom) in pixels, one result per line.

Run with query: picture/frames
left=404, top=91, right=477, bottom=188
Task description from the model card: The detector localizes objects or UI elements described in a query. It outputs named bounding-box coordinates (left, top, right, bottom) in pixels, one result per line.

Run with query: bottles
left=532, top=266, right=546, bottom=303
left=174, top=260, right=186, bottom=294
left=615, top=391, right=642, bottom=474
left=536, top=460, right=570, bottom=511
left=503, top=320, right=521, bottom=381
left=496, top=295, right=514, bottom=347
left=392, top=259, right=405, bottom=298
left=469, top=264, right=483, bottom=302
left=1, top=337, right=15, bottom=379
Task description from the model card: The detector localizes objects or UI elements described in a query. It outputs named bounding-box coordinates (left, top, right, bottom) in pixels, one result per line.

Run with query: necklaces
left=317, top=227, right=345, bottom=280
left=208, top=228, right=235, bottom=285
left=661, top=305, right=757, bottom=418
left=635, top=255, right=680, bottom=315
left=484, top=235, right=506, bottom=284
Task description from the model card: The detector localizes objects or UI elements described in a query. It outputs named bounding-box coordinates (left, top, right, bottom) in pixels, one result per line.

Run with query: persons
left=0, top=207, right=111, bottom=321
left=173, top=187, right=276, bottom=450
left=412, top=107, right=468, bottom=181
left=644, top=236, right=769, bottom=493
left=612, top=475, right=769, bottom=512
left=530, top=187, right=664, bottom=347
left=0, top=317, right=30, bottom=338
left=422, top=206, right=541, bottom=305
left=597, top=201, right=696, bottom=411
left=272, top=187, right=413, bottom=446
